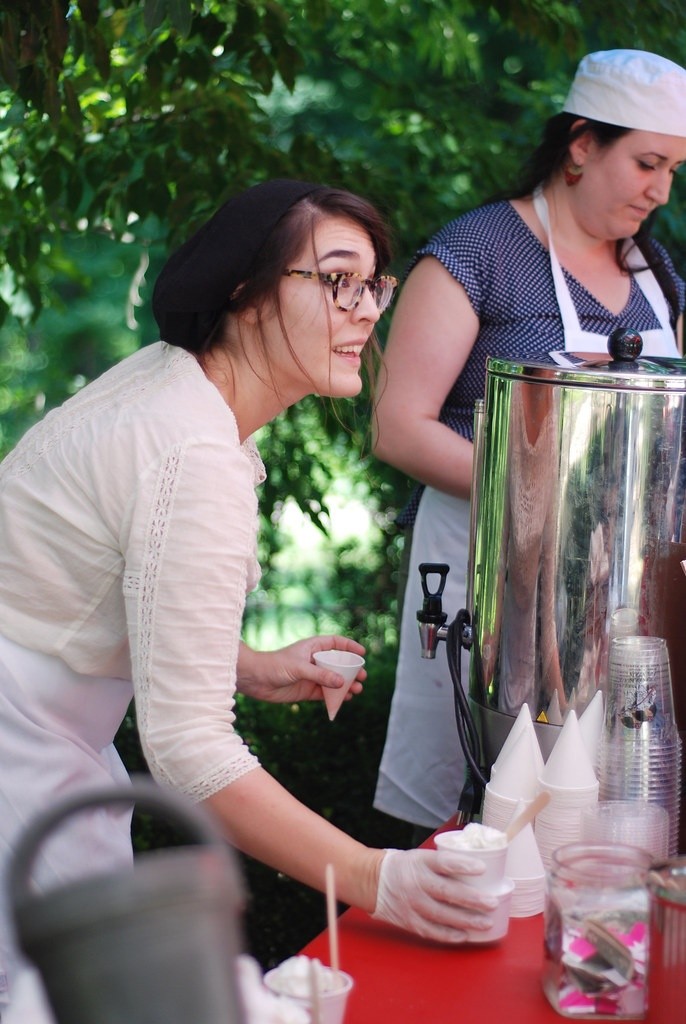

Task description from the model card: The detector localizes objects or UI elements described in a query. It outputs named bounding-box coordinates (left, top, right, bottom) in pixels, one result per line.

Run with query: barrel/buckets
left=6, top=785, right=253, bottom=1023
left=464, top=328, right=684, bottom=788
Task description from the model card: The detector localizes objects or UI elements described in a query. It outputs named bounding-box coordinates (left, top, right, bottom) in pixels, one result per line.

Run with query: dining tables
left=297, top=811, right=685, bottom=1024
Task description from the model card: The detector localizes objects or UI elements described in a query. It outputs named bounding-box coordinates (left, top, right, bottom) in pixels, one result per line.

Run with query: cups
left=538, top=635, right=686, bottom=1023
left=435, top=830, right=514, bottom=945
left=268, top=968, right=353, bottom=1024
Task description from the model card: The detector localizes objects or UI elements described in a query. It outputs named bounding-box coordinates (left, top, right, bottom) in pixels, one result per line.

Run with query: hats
left=560, top=48, right=686, bottom=138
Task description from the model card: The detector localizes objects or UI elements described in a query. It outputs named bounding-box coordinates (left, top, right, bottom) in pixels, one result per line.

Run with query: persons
left=369, top=50, right=685, bottom=828
left=0, top=182, right=499, bottom=1024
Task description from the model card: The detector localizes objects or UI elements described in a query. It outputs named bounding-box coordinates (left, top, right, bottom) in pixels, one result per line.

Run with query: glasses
left=281, top=267, right=400, bottom=312
